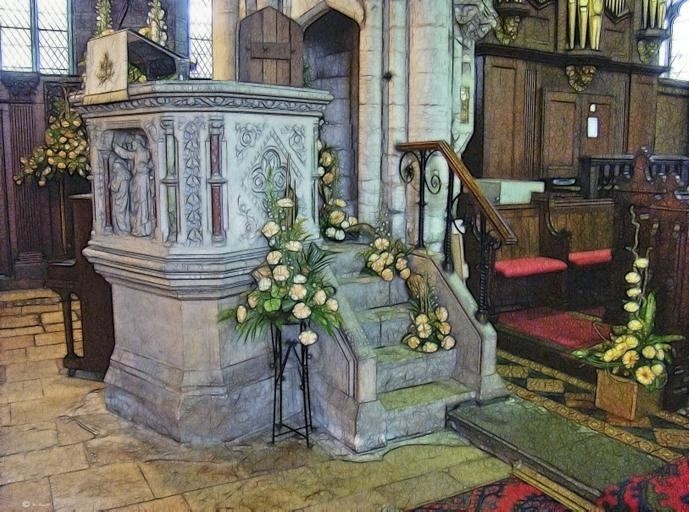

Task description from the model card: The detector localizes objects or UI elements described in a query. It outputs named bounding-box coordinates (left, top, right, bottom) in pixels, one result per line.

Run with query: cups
left=174, top=57, right=191, bottom=82
